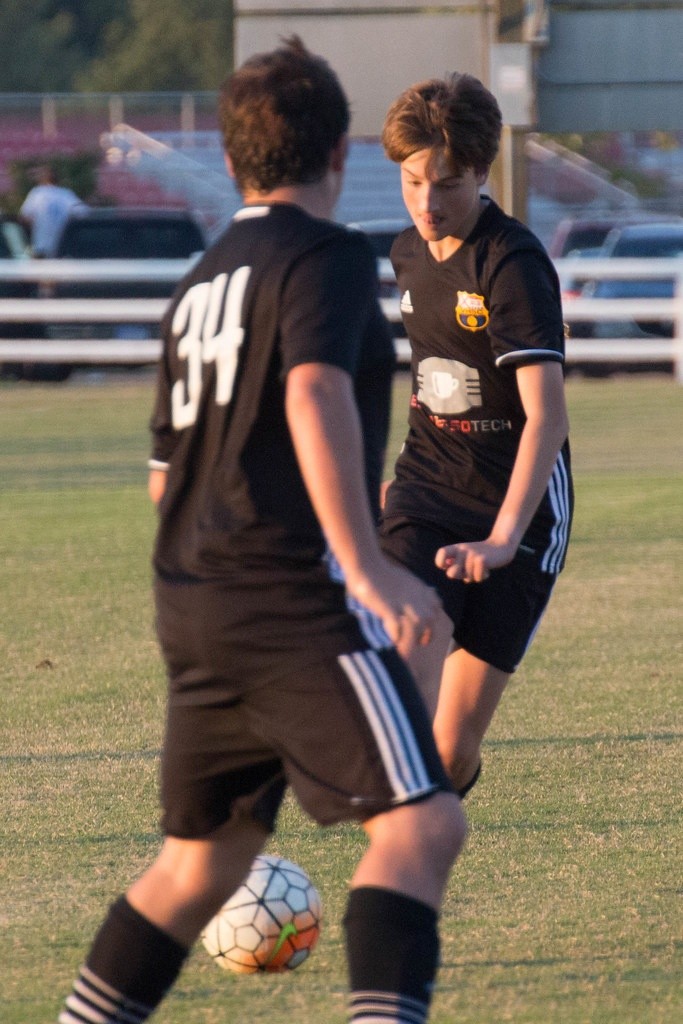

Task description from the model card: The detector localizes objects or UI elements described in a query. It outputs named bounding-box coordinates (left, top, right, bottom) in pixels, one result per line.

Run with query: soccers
left=200, top=854, right=320, bottom=976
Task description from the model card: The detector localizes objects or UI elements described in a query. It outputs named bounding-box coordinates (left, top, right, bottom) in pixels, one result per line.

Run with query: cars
left=551, top=212, right=682, bottom=319
left=42, top=198, right=220, bottom=373
left=568, top=224, right=683, bottom=380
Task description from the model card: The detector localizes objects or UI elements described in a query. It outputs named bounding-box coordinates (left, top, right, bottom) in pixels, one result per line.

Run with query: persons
left=18, top=165, right=96, bottom=298
left=57, top=30, right=469, bottom=1024
left=380, top=76, right=577, bottom=793
